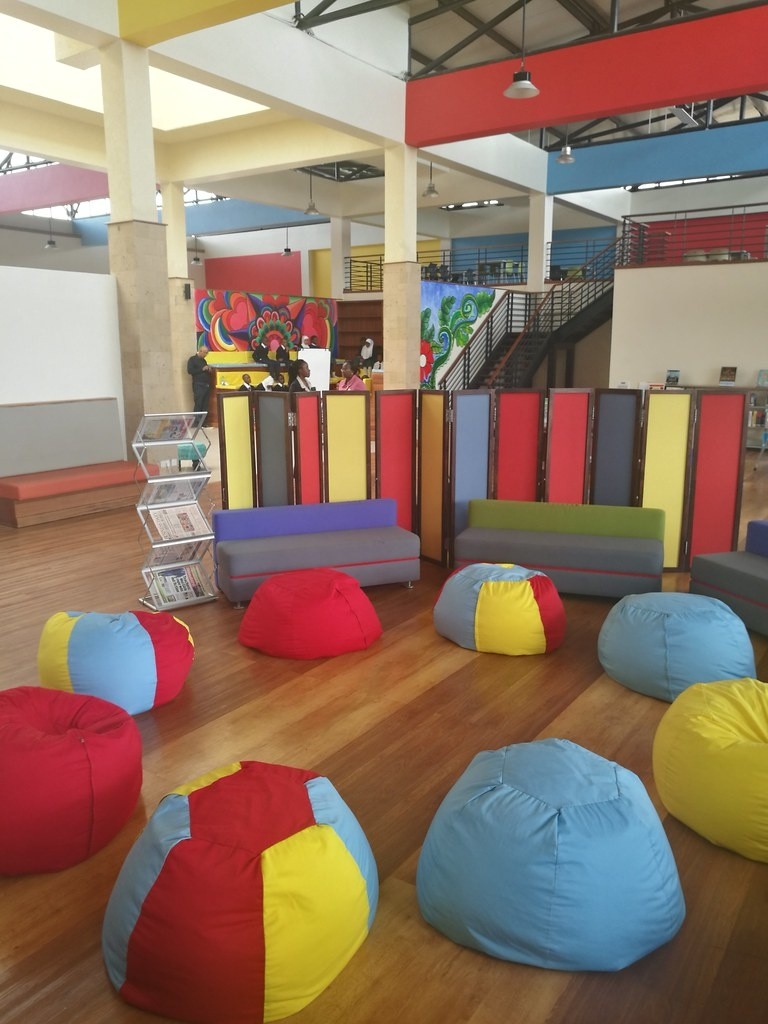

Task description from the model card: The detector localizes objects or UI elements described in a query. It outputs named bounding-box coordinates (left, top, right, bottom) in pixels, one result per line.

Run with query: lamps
left=303, top=169, right=320, bottom=215
left=43, top=208, right=58, bottom=249
left=422, top=161, right=439, bottom=199
left=556, top=122, right=575, bottom=164
left=281, top=227, right=294, bottom=256
left=502, top=0, right=539, bottom=99
left=190, top=237, right=202, bottom=265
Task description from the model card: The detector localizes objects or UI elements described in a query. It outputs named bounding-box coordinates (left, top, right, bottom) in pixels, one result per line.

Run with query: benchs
left=0, top=398, right=159, bottom=528
left=455, top=498, right=665, bottom=598
left=213, top=498, right=420, bottom=609
left=689, top=519, right=768, bottom=637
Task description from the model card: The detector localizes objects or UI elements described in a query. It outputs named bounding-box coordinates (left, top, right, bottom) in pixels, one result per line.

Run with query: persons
left=252, top=337, right=280, bottom=379
left=276, top=338, right=295, bottom=372
left=298, top=335, right=322, bottom=348
left=238, top=373, right=289, bottom=391
left=288, top=359, right=316, bottom=392
left=339, top=360, right=366, bottom=391
left=186, top=344, right=213, bottom=428
left=353, top=337, right=383, bottom=380
